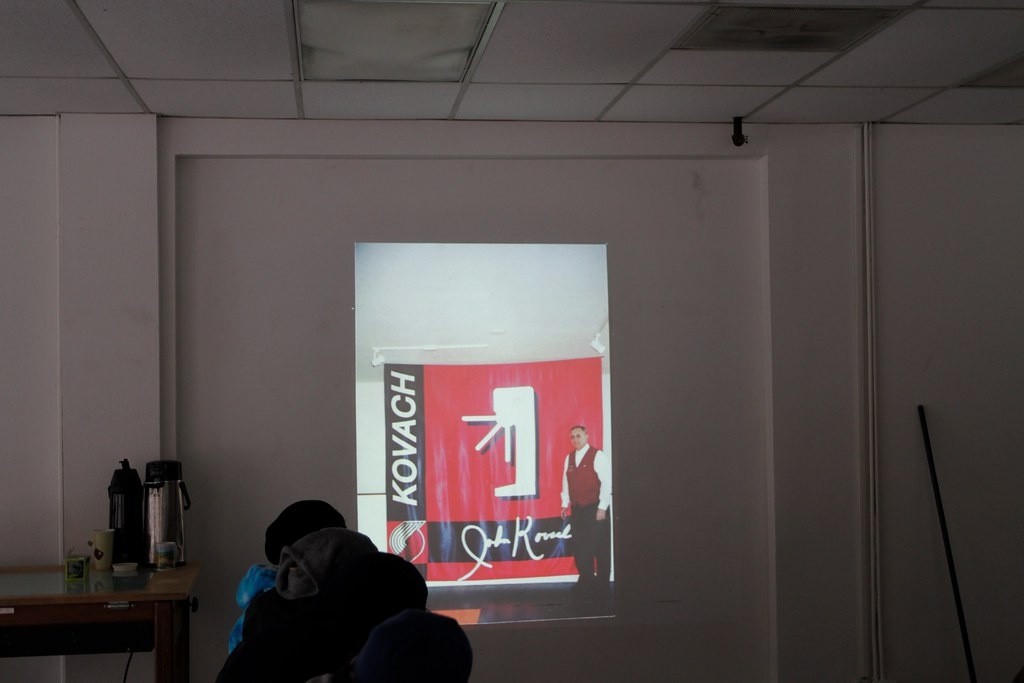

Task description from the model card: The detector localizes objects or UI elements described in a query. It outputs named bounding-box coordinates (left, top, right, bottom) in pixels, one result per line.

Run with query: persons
left=561, top=426, right=612, bottom=601
left=217, top=500, right=473, bottom=683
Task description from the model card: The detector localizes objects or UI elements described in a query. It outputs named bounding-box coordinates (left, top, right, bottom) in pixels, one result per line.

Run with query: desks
left=0, top=559, right=204, bottom=683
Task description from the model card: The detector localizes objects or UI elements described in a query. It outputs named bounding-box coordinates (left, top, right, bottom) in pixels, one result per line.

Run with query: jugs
left=141, top=459, right=191, bottom=569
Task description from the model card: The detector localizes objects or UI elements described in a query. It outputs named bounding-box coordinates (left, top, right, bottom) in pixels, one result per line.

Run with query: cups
left=88, top=529, right=116, bottom=571
left=157, top=541, right=183, bottom=572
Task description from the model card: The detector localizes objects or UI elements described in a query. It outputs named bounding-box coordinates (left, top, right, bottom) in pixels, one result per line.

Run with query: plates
left=112, top=563, right=138, bottom=571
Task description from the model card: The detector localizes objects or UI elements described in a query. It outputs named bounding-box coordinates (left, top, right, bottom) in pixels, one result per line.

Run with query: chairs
left=350, top=610, right=475, bottom=683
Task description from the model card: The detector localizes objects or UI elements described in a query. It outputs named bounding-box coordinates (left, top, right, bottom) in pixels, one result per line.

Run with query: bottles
left=107, top=459, right=143, bottom=563
